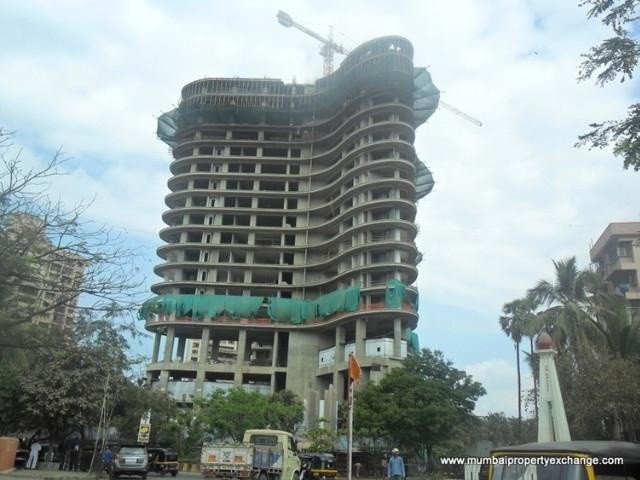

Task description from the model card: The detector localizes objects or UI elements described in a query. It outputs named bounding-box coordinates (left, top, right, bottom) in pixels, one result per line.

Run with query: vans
left=298, top=452, right=337, bottom=478
left=479, top=440, right=640, bottom=480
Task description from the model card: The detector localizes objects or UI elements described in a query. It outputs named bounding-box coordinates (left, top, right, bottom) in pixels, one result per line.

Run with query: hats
left=392, top=448, right=400, bottom=451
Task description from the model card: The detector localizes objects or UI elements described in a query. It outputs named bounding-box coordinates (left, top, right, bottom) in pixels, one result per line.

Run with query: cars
left=112, top=446, right=179, bottom=479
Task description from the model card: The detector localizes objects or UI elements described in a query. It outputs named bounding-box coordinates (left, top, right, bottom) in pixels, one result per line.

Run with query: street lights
left=533, top=331, right=570, bottom=442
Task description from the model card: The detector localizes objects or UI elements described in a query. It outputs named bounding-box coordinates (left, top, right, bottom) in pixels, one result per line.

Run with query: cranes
left=277, top=9, right=483, bottom=128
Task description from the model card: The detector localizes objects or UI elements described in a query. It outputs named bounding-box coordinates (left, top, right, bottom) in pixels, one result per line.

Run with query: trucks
left=200, top=429, right=301, bottom=480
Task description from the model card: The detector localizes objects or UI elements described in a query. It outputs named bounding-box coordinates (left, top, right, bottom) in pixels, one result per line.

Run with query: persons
left=57, top=442, right=66, bottom=471
left=387, top=448, right=406, bottom=479
left=353, top=460, right=362, bottom=478
left=103, top=446, right=112, bottom=473
left=68, top=443, right=79, bottom=472
left=44, top=446, right=55, bottom=469
left=298, top=464, right=310, bottom=480
left=381, top=453, right=388, bottom=477
left=24, top=438, right=43, bottom=470
